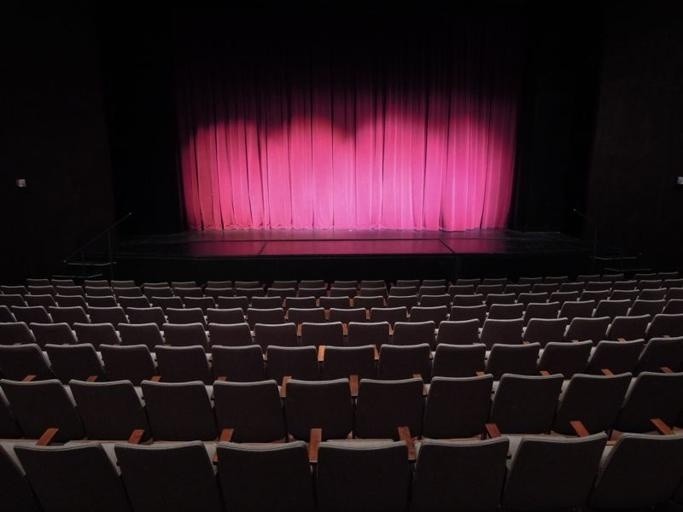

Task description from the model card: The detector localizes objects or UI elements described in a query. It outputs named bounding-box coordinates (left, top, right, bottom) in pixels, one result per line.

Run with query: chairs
left=0, top=270, right=683, bottom=509
